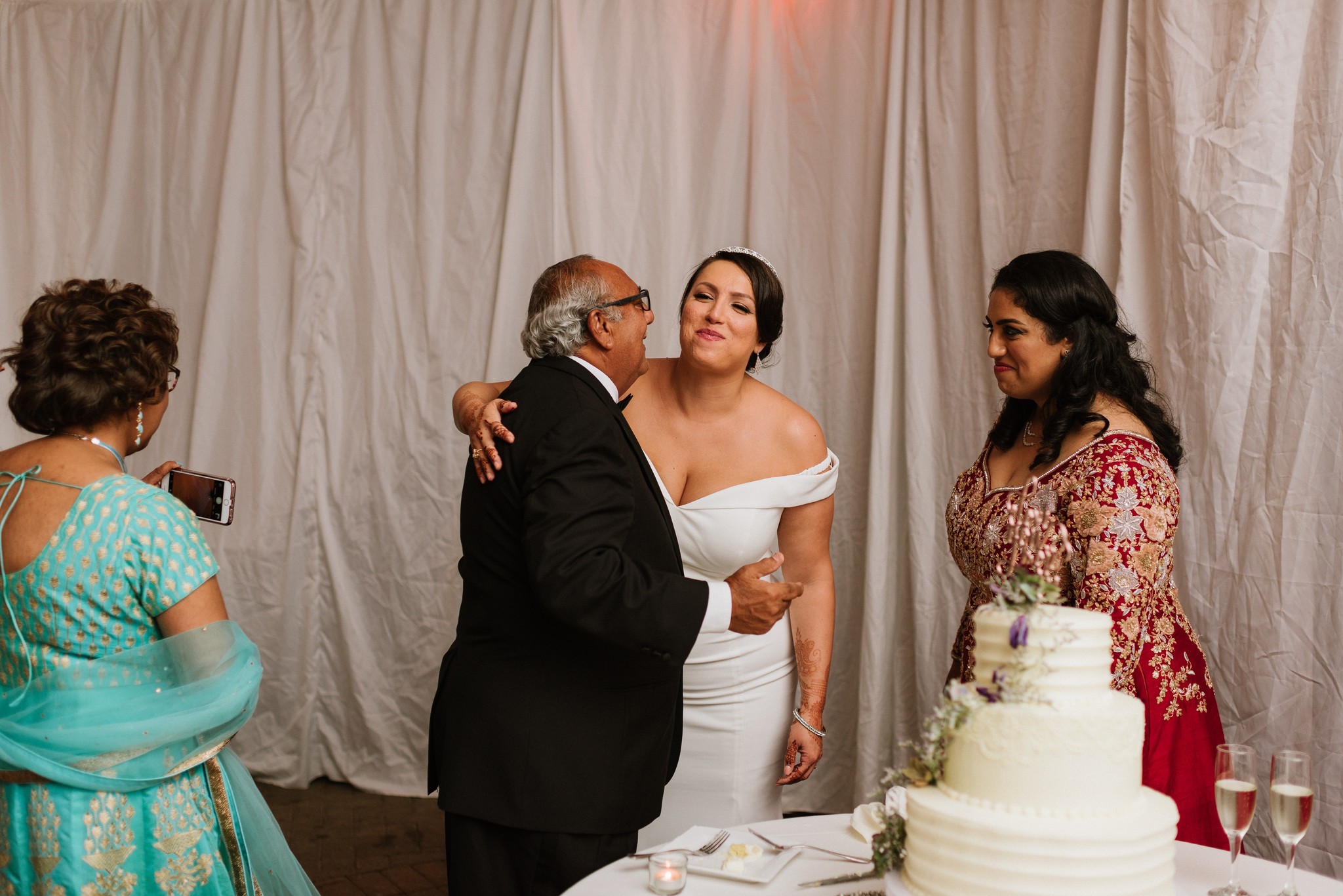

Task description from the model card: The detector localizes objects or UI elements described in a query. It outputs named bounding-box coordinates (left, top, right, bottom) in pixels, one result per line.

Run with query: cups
left=649, top=853, right=687, bottom=896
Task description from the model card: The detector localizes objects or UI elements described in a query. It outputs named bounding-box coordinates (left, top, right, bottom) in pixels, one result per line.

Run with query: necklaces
left=58, top=433, right=128, bottom=474
left=1022, top=397, right=1117, bottom=446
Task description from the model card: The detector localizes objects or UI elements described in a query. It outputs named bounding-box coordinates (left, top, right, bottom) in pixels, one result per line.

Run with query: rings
left=471, top=446, right=484, bottom=459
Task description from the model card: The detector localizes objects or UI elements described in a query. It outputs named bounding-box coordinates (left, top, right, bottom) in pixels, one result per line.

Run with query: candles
left=655, top=870, right=683, bottom=889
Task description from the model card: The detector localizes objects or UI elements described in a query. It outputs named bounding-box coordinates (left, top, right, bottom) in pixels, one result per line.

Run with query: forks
left=627, top=829, right=731, bottom=857
left=747, top=827, right=872, bottom=864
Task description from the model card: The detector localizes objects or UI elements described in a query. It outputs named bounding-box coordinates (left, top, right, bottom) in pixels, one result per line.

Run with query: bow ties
left=616, top=393, right=634, bottom=412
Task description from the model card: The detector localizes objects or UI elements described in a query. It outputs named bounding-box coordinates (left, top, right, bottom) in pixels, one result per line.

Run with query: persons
left=425, top=252, right=803, bottom=896
left=450, top=244, right=839, bottom=855
left=0, top=274, right=321, bottom=896
left=941, top=250, right=1249, bottom=859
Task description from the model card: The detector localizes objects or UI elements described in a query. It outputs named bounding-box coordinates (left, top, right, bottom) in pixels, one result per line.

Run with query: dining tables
left=560, top=808, right=1342, bottom=896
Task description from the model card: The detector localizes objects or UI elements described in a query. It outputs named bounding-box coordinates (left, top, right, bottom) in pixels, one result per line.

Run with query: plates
left=653, top=824, right=802, bottom=883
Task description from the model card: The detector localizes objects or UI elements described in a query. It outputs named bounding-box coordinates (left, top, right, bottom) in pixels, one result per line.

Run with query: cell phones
left=160, top=466, right=236, bottom=526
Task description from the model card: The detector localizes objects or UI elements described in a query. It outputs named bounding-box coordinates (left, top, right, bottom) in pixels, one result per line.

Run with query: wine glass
left=1270, top=750, right=1313, bottom=896
left=1206, top=744, right=1258, bottom=896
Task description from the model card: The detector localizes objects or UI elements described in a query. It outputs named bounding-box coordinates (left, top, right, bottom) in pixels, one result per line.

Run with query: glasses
left=597, top=288, right=651, bottom=312
left=166, top=364, right=180, bottom=392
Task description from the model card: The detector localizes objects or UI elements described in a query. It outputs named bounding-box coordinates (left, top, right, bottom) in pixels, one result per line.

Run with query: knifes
left=797, top=869, right=881, bottom=888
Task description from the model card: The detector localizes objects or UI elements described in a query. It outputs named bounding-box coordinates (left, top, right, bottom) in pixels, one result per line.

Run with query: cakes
left=889, top=595, right=1182, bottom=896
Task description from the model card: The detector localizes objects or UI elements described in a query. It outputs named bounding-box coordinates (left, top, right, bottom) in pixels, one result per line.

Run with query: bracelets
left=792, top=706, right=828, bottom=738
